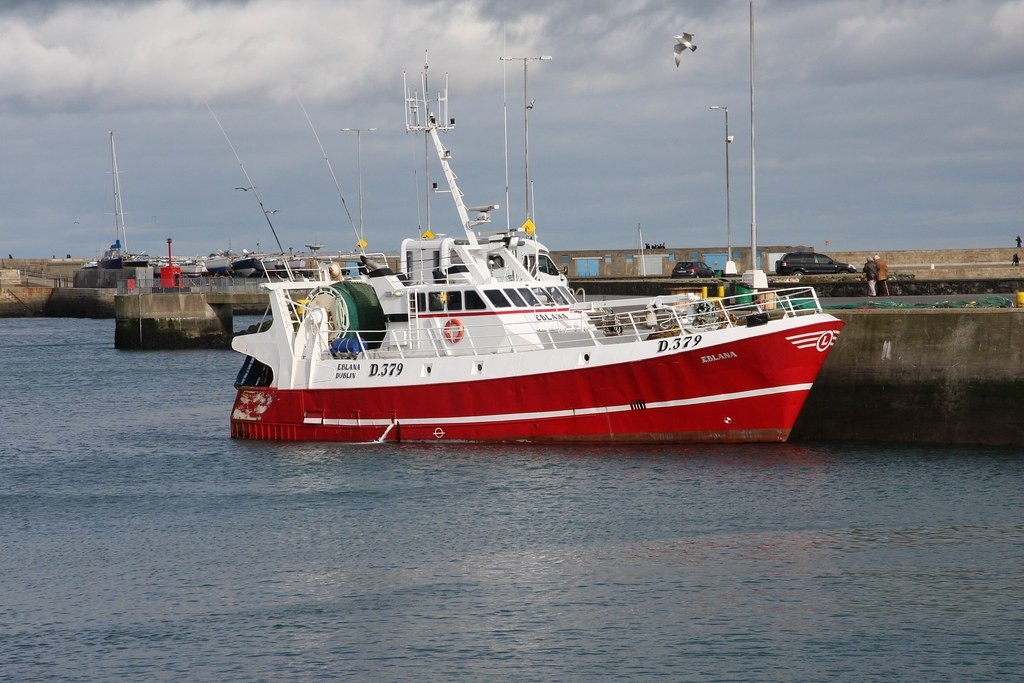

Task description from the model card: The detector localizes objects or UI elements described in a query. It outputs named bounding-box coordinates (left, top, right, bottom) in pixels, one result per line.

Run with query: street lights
left=340, top=127, right=378, bottom=240
left=499, top=55, right=552, bottom=219
left=708, top=105, right=734, bottom=261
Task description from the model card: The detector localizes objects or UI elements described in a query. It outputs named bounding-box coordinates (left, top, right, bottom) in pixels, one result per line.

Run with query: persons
left=1012, top=252, right=1019, bottom=266
left=1015, top=236, right=1022, bottom=249
left=863, top=255, right=890, bottom=297
left=9, top=254, right=13, bottom=259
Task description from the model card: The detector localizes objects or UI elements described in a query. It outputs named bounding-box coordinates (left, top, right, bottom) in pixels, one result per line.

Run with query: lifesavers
left=443, top=320, right=464, bottom=344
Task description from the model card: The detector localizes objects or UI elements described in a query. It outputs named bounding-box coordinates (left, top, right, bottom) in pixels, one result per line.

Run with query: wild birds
left=672, top=32, right=697, bottom=68
left=234, top=186, right=257, bottom=193
left=73, top=220, right=80, bottom=225
left=264, top=209, right=284, bottom=215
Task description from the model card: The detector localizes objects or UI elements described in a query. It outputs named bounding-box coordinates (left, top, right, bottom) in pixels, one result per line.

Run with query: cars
left=671, top=260, right=715, bottom=278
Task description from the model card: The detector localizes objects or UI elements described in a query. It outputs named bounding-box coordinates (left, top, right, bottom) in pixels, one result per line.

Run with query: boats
left=82, top=129, right=314, bottom=277
left=198, top=51, right=848, bottom=444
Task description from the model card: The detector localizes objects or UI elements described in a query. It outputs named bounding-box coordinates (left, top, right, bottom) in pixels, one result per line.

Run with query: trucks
left=399, top=232, right=570, bottom=305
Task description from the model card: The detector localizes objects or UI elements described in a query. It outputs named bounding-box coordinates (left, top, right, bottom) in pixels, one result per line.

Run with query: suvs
left=775, top=250, right=858, bottom=276
